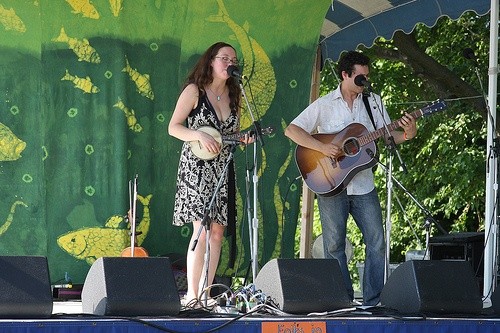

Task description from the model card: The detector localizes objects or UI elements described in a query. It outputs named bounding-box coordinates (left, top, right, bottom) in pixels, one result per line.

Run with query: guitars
left=189, top=125, right=276, bottom=160
left=294, top=99, right=447, bottom=198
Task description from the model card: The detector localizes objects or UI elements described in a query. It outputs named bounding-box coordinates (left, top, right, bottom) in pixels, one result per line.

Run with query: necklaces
left=207, top=81, right=226, bottom=101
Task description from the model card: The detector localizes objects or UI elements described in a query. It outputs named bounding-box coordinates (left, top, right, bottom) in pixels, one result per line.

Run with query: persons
left=284, top=50, right=418, bottom=306
left=168, top=41, right=253, bottom=308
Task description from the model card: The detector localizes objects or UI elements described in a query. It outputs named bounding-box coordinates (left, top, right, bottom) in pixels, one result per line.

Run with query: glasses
left=212, top=56, right=237, bottom=64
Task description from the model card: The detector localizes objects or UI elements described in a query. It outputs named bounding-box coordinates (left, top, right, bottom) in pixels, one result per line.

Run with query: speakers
left=0, top=256, right=53, bottom=319
left=80, top=257, right=180, bottom=316
left=491, top=284, right=500, bottom=311
left=250, top=257, right=350, bottom=312
left=378, top=260, right=483, bottom=313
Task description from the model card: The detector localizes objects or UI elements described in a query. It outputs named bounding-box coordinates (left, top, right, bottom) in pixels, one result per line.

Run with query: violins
left=121, top=209, right=149, bottom=257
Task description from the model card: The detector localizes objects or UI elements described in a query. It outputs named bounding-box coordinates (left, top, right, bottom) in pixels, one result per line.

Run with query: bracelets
left=404, top=131, right=409, bottom=140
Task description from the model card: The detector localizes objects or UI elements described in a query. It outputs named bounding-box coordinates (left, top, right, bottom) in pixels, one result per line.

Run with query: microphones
left=353, top=74, right=368, bottom=86
left=227, top=65, right=247, bottom=81
left=222, top=139, right=245, bottom=146
left=365, top=148, right=374, bottom=157
